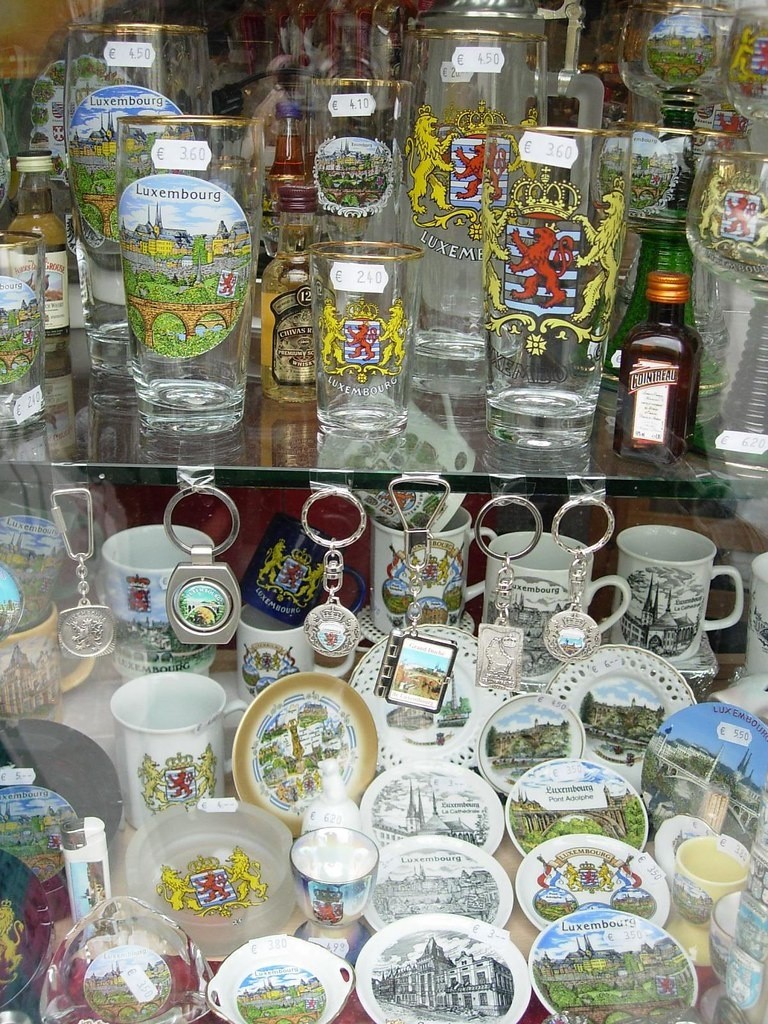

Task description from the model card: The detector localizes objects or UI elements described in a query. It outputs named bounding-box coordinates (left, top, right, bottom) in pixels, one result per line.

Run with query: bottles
left=263, top=102, right=308, bottom=258
left=261, top=186, right=319, bottom=402
left=613, top=271, right=703, bottom=466
left=6, top=151, right=70, bottom=353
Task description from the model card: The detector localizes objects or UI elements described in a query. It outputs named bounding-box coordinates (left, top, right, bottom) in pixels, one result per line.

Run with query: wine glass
left=290, top=827, right=379, bottom=967
left=700, top=891, right=742, bottom=1024
left=667, top=836, right=749, bottom=966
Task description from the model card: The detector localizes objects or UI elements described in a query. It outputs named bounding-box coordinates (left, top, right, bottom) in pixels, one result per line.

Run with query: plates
left=0, top=849, right=53, bottom=1008
left=232, top=672, right=378, bottom=838
left=515, top=834, right=671, bottom=932
left=206, top=935, right=356, bottom=1024
left=359, top=762, right=504, bottom=856
left=505, top=758, right=649, bottom=858
left=348, top=624, right=697, bottom=796
left=641, top=702, right=767, bottom=854
left=528, top=909, right=698, bottom=1024
left=42, top=895, right=217, bottom=1024
left=125, top=802, right=294, bottom=956
left=355, top=913, right=531, bottom=1024
left=364, top=835, right=514, bottom=932
left=0, top=720, right=123, bottom=894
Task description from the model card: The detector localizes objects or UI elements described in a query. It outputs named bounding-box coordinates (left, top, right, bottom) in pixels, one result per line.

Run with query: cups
left=310, top=79, right=413, bottom=244
left=0, top=484, right=105, bottom=633
left=0, top=600, right=95, bottom=723
left=370, top=505, right=498, bottom=636
left=101, top=525, right=217, bottom=685
left=482, top=524, right=768, bottom=682
left=354, top=491, right=466, bottom=533
left=110, top=672, right=248, bottom=832
left=398, top=30, right=547, bottom=397
left=726, top=772, right=768, bottom=1024
left=309, top=242, right=424, bottom=442
left=237, top=604, right=356, bottom=705
left=484, top=125, right=632, bottom=450
left=117, top=115, right=264, bottom=435
left=65, top=23, right=213, bottom=380
left=240, top=513, right=366, bottom=627
left=0, top=231, right=44, bottom=440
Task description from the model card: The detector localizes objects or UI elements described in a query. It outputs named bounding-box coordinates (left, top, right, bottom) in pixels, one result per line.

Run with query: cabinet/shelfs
left=0, top=387, right=768, bottom=1024
